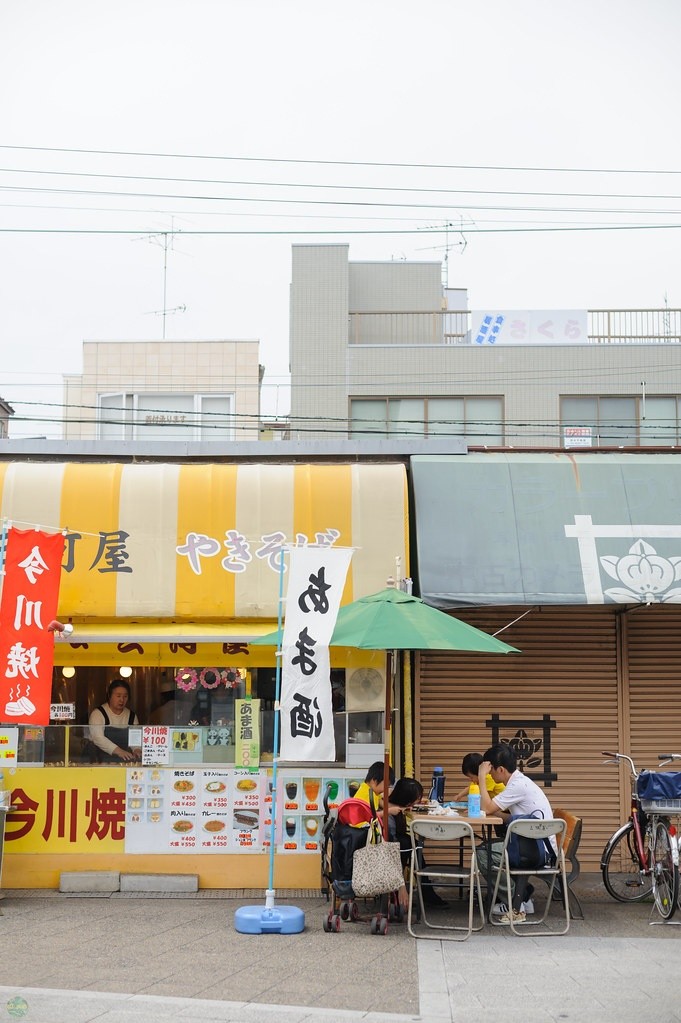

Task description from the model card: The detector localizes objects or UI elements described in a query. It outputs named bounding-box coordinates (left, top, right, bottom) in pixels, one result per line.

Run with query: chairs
left=406, top=819, right=485, bottom=940
left=488, top=818, right=571, bottom=936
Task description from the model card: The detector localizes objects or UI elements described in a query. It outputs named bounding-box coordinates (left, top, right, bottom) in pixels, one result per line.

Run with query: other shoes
left=418, top=891, right=451, bottom=908
left=512, top=881, right=534, bottom=911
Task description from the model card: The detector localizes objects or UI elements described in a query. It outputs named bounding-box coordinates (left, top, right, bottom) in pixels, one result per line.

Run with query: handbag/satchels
left=351, top=827, right=405, bottom=897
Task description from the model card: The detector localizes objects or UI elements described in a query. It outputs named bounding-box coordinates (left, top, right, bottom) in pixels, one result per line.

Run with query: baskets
left=641, top=798, right=681, bottom=811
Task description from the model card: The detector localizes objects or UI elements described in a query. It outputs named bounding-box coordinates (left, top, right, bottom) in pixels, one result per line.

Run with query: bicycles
left=600, top=749, right=681, bottom=927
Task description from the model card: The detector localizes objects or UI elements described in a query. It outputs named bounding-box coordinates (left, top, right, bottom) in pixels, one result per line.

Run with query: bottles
left=430, top=767, right=446, bottom=806
left=468, top=785, right=481, bottom=818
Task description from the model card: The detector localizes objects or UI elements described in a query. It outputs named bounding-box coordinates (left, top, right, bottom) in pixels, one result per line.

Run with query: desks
left=403, top=803, right=503, bottom=924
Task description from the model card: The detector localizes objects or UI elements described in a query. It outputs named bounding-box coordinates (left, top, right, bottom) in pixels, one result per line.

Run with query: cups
left=304, top=818, right=318, bottom=837
left=349, top=781, right=360, bottom=797
left=326, top=781, right=339, bottom=800
left=286, top=783, right=297, bottom=799
left=286, top=818, right=296, bottom=838
left=306, top=781, right=319, bottom=802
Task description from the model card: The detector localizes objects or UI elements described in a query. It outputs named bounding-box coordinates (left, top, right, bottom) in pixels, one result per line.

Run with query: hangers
left=176, top=667, right=241, bottom=693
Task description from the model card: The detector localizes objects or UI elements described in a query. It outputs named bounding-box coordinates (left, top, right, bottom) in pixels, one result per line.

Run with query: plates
left=171, top=779, right=195, bottom=793
left=235, top=779, right=258, bottom=793
left=442, top=802, right=467, bottom=809
left=232, top=811, right=260, bottom=829
left=202, top=820, right=226, bottom=833
left=171, top=819, right=195, bottom=833
left=203, top=781, right=226, bottom=793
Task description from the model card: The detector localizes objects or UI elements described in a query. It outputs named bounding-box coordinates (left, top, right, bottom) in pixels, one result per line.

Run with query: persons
left=478, top=743, right=558, bottom=916
left=451, top=753, right=512, bottom=837
left=83, top=680, right=143, bottom=763
left=352, top=761, right=442, bottom=916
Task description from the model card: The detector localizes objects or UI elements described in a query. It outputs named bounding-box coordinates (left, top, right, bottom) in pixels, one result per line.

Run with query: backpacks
left=505, top=809, right=560, bottom=870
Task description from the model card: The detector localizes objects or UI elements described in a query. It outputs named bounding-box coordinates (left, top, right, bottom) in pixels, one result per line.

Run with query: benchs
left=512, top=810, right=583, bottom=920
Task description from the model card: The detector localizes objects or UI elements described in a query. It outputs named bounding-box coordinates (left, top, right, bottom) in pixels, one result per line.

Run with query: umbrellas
left=249, top=587, right=522, bottom=840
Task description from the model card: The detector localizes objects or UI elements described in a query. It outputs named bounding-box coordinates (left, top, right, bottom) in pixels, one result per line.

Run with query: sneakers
left=498, top=908, right=526, bottom=923
left=523, top=898, right=535, bottom=914
left=492, top=901, right=525, bottom=915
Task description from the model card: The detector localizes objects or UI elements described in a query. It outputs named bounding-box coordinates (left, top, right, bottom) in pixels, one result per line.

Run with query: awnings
left=0, top=461, right=408, bottom=644
left=411, top=453, right=681, bottom=608
